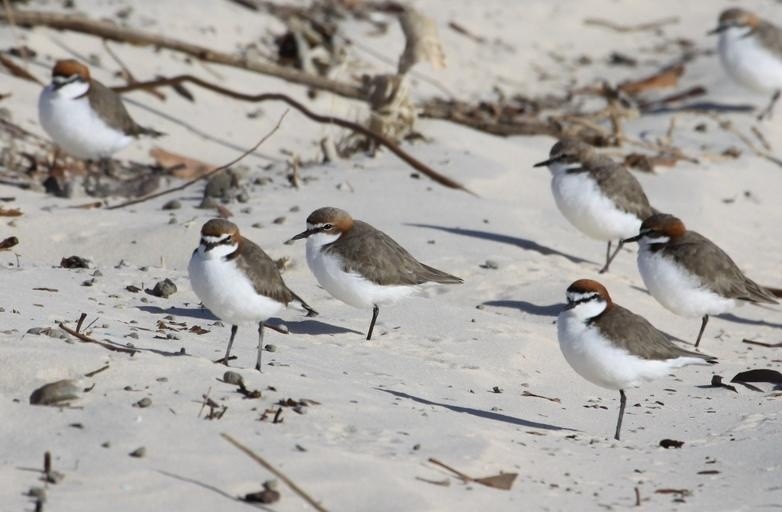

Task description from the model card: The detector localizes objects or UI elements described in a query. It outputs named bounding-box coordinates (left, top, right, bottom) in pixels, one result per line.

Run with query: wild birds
left=554, top=277, right=718, bottom=434
left=37, top=51, right=169, bottom=192
left=282, top=204, right=465, bottom=340
left=704, top=4, right=782, bottom=120
left=621, top=212, right=782, bottom=349
left=531, top=134, right=661, bottom=273
left=187, top=217, right=321, bottom=366
